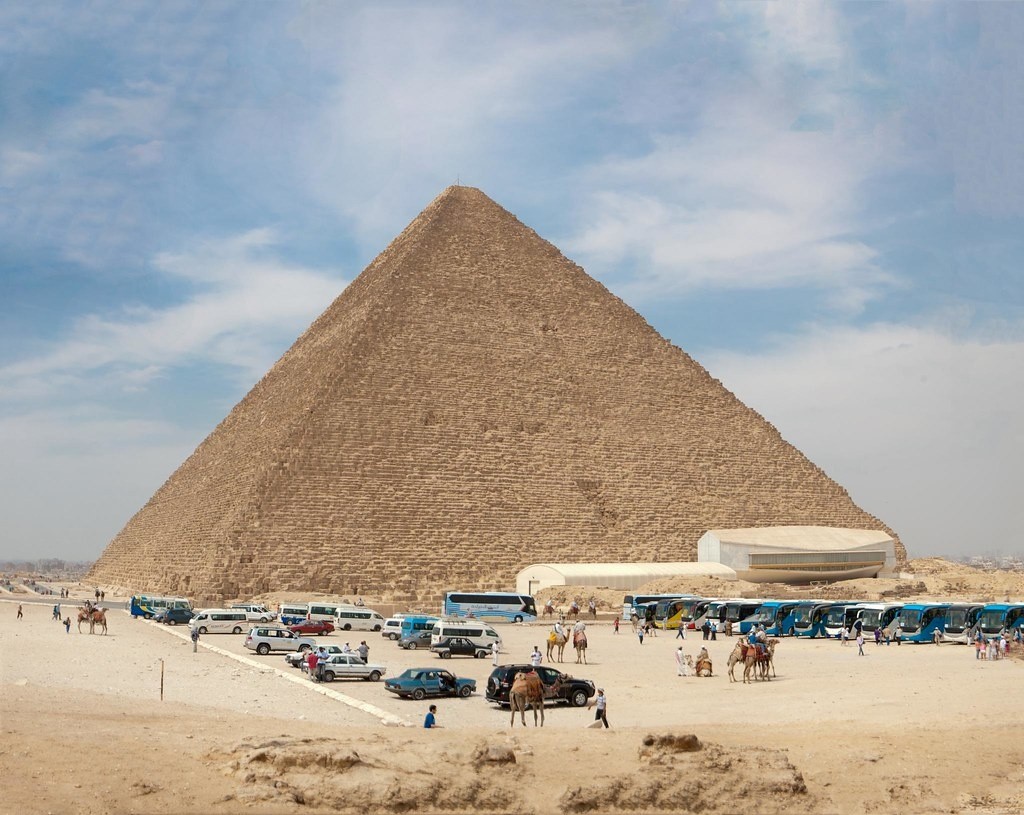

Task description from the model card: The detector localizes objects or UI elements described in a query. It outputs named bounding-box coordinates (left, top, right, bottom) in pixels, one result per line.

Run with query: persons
left=725, top=619, right=732, bottom=636
left=307, top=647, right=329, bottom=683
left=16, top=579, right=105, bottom=635
left=613, top=617, right=619, bottom=634
left=531, top=646, right=542, bottom=666
left=702, top=619, right=717, bottom=640
left=423, top=704, right=444, bottom=728
left=930, top=627, right=941, bottom=646
left=190, top=627, right=200, bottom=652
left=588, top=688, right=609, bottom=728
left=874, top=626, right=902, bottom=645
left=675, top=646, right=708, bottom=676
left=572, top=600, right=577, bottom=608
left=966, top=626, right=1024, bottom=661
left=572, top=619, right=588, bottom=648
left=630, top=612, right=688, bottom=644
left=492, top=640, right=499, bottom=666
left=553, top=621, right=564, bottom=640
left=857, top=633, right=865, bottom=655
left=547, top=600, right=553, bottom=607
left=747, top=622, right=768, bottom=655
left=343, top=640, right=370, bottom=665
left=841, top=625, right=849, bottom=646
left=590, top=601, right=595, bottom=609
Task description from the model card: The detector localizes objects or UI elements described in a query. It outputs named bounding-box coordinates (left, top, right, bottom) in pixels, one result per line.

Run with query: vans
left=306, top=602, right=355, bottom=624
left=400, top=617, right=441, bottom=640
left=230, top=604, right=277, bottom=624
left=187, top=608, right=250, bottom=634
left=431, top=621, right=502, bottom=650
left=393, top=612, right=433, bottom=618
left=334, top=607, right=386, bottom=632
left=381, top=619, right=404, bottom=640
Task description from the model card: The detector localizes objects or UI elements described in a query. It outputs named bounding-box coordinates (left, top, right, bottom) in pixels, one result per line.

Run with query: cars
left=302, top=653, right=387, bottom=683
left=284, top=645, right=343, bottom=668
left=153, top=609, right=195, bottom=626
left=430, top=636, right=498, bottom=659
left=397, top=631, right=433, bottom=651
left=384, top=667, right=477, bottom=700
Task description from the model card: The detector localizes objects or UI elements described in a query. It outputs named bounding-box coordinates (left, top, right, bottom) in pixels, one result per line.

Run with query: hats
left=598, top=688, right=604, bottom=694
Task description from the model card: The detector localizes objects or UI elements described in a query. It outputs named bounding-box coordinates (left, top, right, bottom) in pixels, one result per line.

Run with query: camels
left=727, top=639, right=779, bottom=685
left=546, top=627, right=571, bottom=664
left=509, top=671, right=573, bottom=728
left=77, top=606, right=110, bottom=635
left=629, top=612, right=638, bottom=634
left=567, top=605, right=582, bottom=620
left=696, top=654, right=712, bottom=678
left=541, top=605, right=555, bottom=619
left=573, top=629, right=588, bottom=665
left=588, top=605, right=597, bottom=618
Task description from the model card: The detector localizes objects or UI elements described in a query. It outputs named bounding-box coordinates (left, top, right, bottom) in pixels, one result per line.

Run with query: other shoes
left=493, top=664, right=497, bottom=666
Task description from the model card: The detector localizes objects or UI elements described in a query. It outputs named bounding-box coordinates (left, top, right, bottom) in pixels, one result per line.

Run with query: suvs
left=485, top=664, right=596, bottom=711
left=243, top=625, right=316, bottom=655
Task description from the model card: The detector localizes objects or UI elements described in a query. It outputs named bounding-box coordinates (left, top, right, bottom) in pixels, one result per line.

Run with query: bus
left=621, top=594, right=885, bottom=641
left=130, top=594, right=192, bottom=619
left=276, top=604, right=308, bottom=625
left=441, top=591, right=538, bottom=624
left=857, top=603, right=906, bottom=641
left=975, top=604, right=1024, bottom=643
left=894, top=603, right=955, bottom=644
left=943, top=604, right=988, bottom=646
